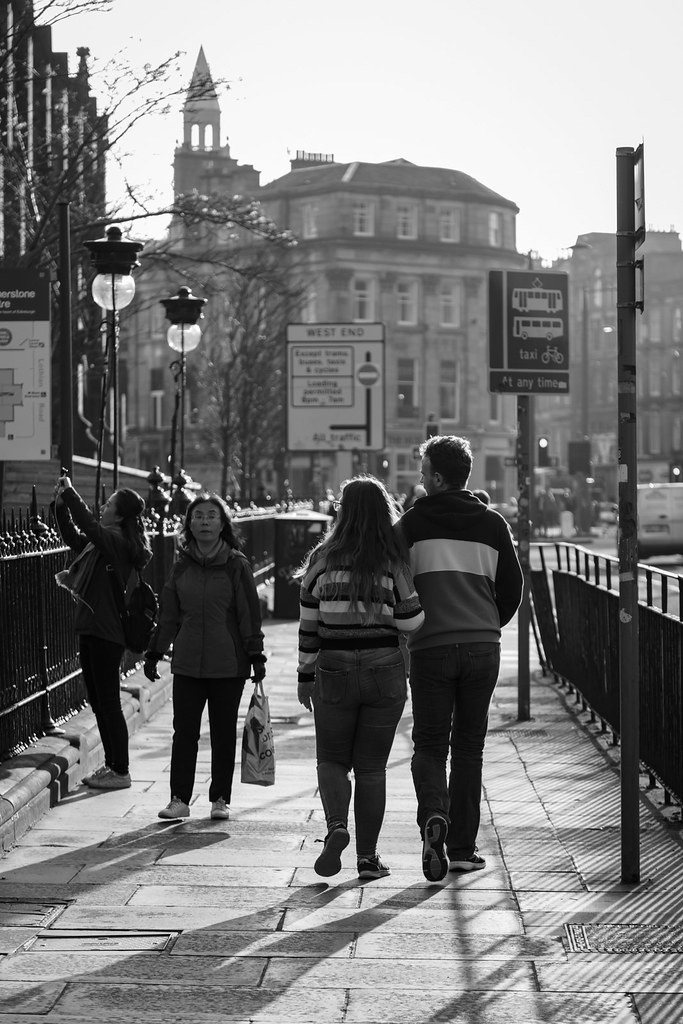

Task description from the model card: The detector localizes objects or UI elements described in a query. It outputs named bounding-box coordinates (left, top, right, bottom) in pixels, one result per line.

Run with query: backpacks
left=103, top=547, right=158, bottom=653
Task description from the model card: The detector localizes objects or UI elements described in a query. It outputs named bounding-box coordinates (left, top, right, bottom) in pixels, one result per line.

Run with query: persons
left=472, top=490, right=490, bottom=507
left=51, top=477, right=152, bottom=788
left=297, top=479, right=425, bottom=880
left=402, top=484, right=428, bottom=512
left=143, top=493, right=267, bottom=820
left=507, top=489, right=549, bottom=538
left=253, top=487, right=277, bottom=507
left=390, top=436, right=523, bottom=882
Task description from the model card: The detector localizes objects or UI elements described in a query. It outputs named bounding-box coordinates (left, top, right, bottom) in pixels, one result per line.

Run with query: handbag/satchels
left=241, top=678, right=276, bottom=787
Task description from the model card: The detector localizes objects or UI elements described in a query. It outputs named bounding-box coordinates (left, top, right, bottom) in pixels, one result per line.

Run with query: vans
left=610, top=482, right=683, bottom=560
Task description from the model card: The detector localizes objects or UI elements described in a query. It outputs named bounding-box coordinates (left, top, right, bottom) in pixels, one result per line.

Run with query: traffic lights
left=669, top=464, right=683, bottom=483
left=534, top=434, right=559, bottom=468
left=425, top=421, right=440, bottom=441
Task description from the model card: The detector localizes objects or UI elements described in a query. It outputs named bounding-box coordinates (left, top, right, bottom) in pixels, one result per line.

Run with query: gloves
left=143, top=652, right=162, bottom=682
left=250, top=655, right=267, bottom=684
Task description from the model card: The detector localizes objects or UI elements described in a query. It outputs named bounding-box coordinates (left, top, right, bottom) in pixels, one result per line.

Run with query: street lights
left=157, top=285, right=210, bottom=472
left=82, top=224, right=147, bottom=494
left=525, top=243, right=591, bottom=539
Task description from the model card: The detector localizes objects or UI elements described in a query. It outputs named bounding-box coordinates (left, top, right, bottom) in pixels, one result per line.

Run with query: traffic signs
left=282, top=320, right=388, bottom=453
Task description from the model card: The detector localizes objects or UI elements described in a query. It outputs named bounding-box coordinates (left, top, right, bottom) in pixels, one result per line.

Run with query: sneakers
left=311, top=823, right=349, bottom=877
left=89, top=770, right=131, bottom=789
left=158, top=796, right=190, bottom=819
left=421, top=814, right=448, bottom=881
left=449, top=849, right=486, bottom=871
left=81, top=765, right=109, bottom=783
left=210, top=798, right=232, bottom=819
left=358, top=853, right=391, bottom=879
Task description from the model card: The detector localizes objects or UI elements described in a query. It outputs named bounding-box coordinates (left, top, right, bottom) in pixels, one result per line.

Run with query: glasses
left=188, top=511, right=221, bottom=525
left=332, top=500, right=346, bottom=510
